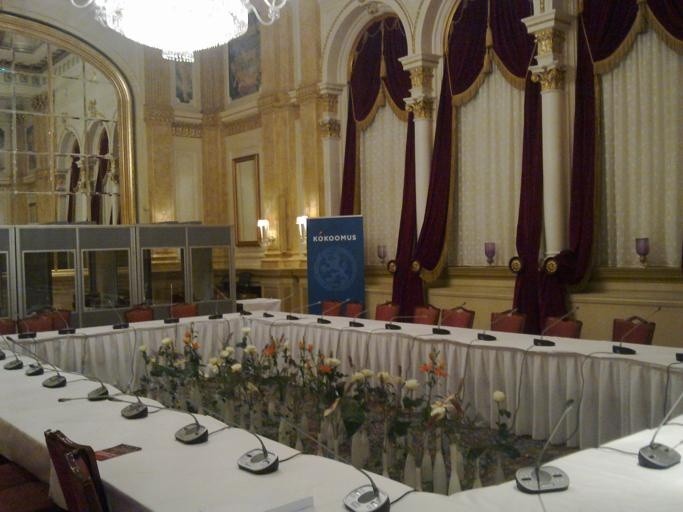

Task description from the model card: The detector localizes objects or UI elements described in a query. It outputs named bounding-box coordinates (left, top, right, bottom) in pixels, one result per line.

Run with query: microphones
left=0, top=283, right=683, bottom=511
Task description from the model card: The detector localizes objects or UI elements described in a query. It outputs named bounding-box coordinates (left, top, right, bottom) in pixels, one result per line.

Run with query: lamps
left=257, top=220, right=270, bottom=245
left=295, top=216, right=308, bottom=240
left=67, top=0, right=287, bottom=64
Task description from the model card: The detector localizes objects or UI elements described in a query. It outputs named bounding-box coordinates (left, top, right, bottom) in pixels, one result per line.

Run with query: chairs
left=0, top=298, right=683, bottom=512
